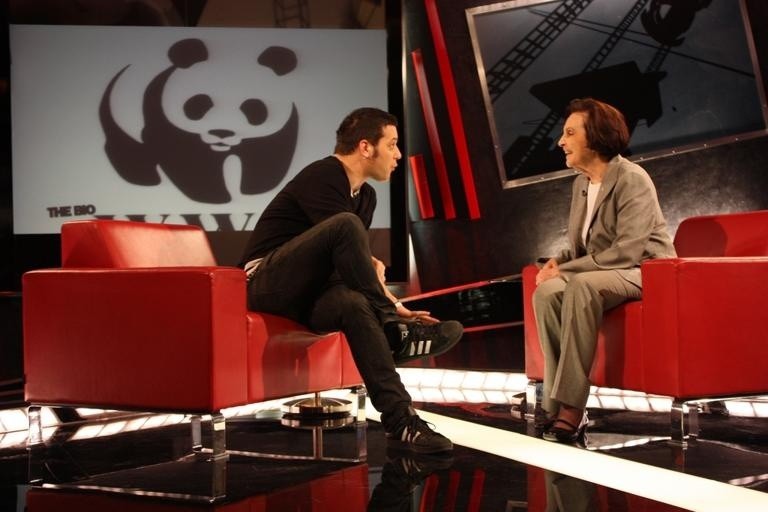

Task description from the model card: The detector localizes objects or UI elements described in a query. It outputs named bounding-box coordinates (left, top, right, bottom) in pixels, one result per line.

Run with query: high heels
left=535, top=411, right=590, bottom=446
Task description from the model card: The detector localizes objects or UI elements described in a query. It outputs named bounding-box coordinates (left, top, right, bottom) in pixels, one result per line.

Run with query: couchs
left=22, top=219, right=368, bottom=505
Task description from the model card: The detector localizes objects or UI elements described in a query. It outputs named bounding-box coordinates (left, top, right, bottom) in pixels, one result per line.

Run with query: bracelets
left=392, top=299, right=403, bottom=307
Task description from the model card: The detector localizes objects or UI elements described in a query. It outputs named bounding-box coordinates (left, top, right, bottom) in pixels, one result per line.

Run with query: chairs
left=522, top=210, right=768, bottom=451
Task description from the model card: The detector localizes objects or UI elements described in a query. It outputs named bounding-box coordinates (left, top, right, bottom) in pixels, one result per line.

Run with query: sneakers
left=384, top=418, right=455, bottom=454
left=391, top=318, right=464, bottom=364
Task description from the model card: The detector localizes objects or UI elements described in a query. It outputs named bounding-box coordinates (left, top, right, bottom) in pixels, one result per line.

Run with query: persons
left=366, top=454, right=457, bottom=512
left=525, top=94, right=676, bottom=443
left=236, top=104, right=464, bottom=453
